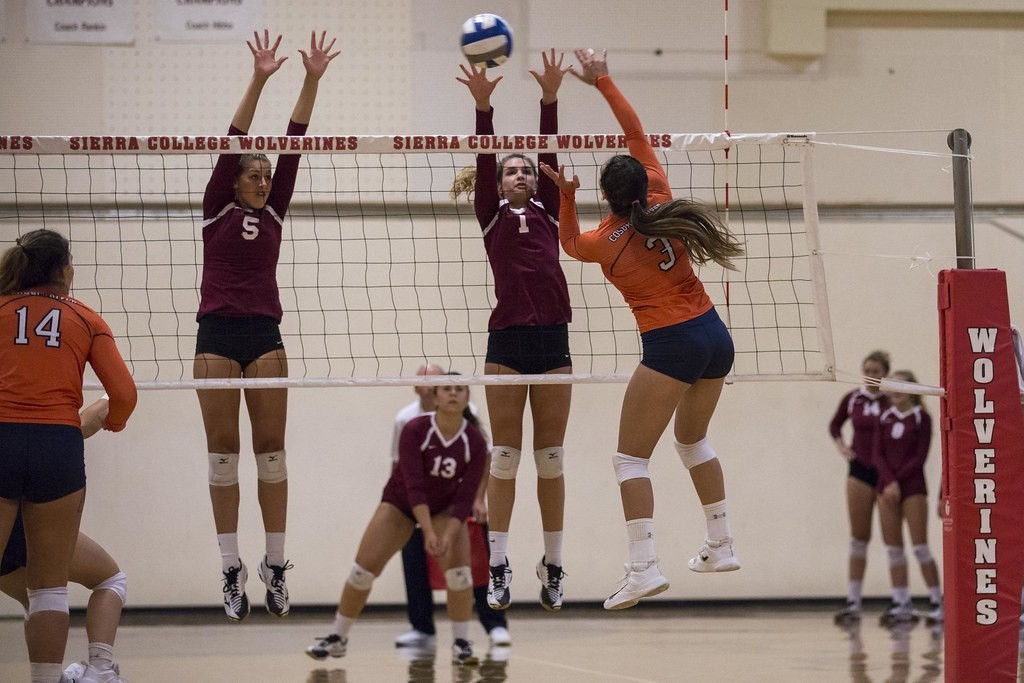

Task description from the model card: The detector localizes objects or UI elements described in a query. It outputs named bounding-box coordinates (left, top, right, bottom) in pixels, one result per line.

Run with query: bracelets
left=100, top=393, right=109, bottom=400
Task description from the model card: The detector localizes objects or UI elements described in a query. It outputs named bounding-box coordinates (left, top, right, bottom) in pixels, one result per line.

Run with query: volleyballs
left=460, top=12, right=514, bottom=68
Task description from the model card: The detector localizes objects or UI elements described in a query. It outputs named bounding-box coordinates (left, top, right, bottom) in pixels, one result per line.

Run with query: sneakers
left=486, top=556, right=514, bottom=610
left=689, top=537, right=741, bottom=573
left=486, top=628, right=512, bottom=644
left=603, top=563, right=670, bottom=610
left=257, top=555, right=293, bottom=617
left=536, top=555, right=568, bottom=612
left=393, top=630, right=438, bottom=646
left=79, top=661, right=126, bottom=683
left=222, top=558, right=250, bottom=622
left=64, top=663, right=84, bottom=683
left=306, top=634, right=348, bottom=659
left=452, top=638, right=480, bottom=662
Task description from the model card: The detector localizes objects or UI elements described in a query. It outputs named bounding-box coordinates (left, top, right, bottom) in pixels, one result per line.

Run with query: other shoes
left=834, top=601, right=862, bottom=613
left=926, top=600, right=941, bottom=620
left=884, top=601, right=910, bottom=617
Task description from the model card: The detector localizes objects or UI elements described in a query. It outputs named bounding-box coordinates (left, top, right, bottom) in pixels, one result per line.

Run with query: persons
left=829, top=351, right=895, bottom=624
left=0, top=229, right=140, bottom=683
left=292, top=363, right=525, bottom=662
left=192, top=28, right=343, bottom=623
left=536, top=41, right=741, bottom=612
left=873, top=370, right=944, bottom=628
left=0, top=382, right=129, bottom=683
left=456, top=47, right=575, bottom=612
